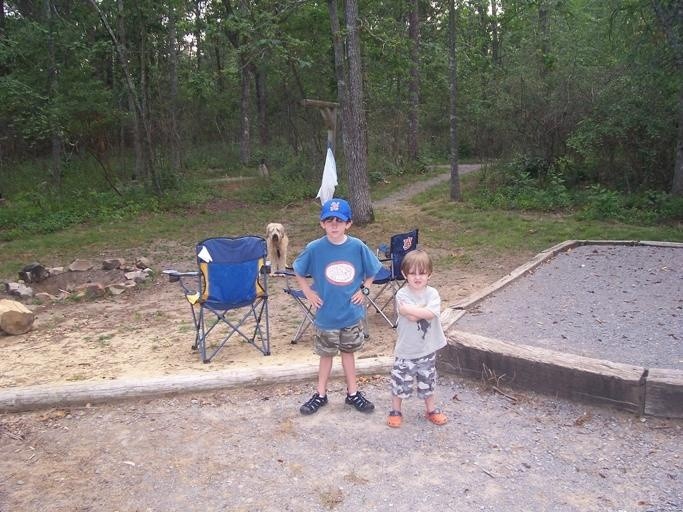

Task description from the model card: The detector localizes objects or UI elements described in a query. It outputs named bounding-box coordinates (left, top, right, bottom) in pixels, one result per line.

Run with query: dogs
left=265, top=222, right=289, bottom=276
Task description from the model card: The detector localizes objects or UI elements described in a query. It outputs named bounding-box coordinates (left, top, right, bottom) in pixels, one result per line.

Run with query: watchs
left=359, top=283, right=370, bottom=296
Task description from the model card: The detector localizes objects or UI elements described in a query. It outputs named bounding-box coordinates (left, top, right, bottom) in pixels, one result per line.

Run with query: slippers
left=429, top=408, right=447, bottom=424
left=388, top=411, right=402, bottom=426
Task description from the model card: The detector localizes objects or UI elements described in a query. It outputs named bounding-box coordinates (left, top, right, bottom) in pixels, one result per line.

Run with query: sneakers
left=300, top=393, right=327, bottom=415
left=345, top=391, right=374, bottom=410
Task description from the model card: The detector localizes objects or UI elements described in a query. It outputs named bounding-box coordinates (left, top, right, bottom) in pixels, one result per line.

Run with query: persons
left=293, top=198, right=384, bottom=414
left=386, top=249, right=449, bottom=428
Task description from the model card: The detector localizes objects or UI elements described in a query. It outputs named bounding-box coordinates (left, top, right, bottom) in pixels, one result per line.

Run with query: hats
left=320, top=199, right=352, bottom=221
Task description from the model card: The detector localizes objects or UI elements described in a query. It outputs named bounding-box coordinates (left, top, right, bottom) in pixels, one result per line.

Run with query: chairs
left=160, top=227, right=423, bottom=365
